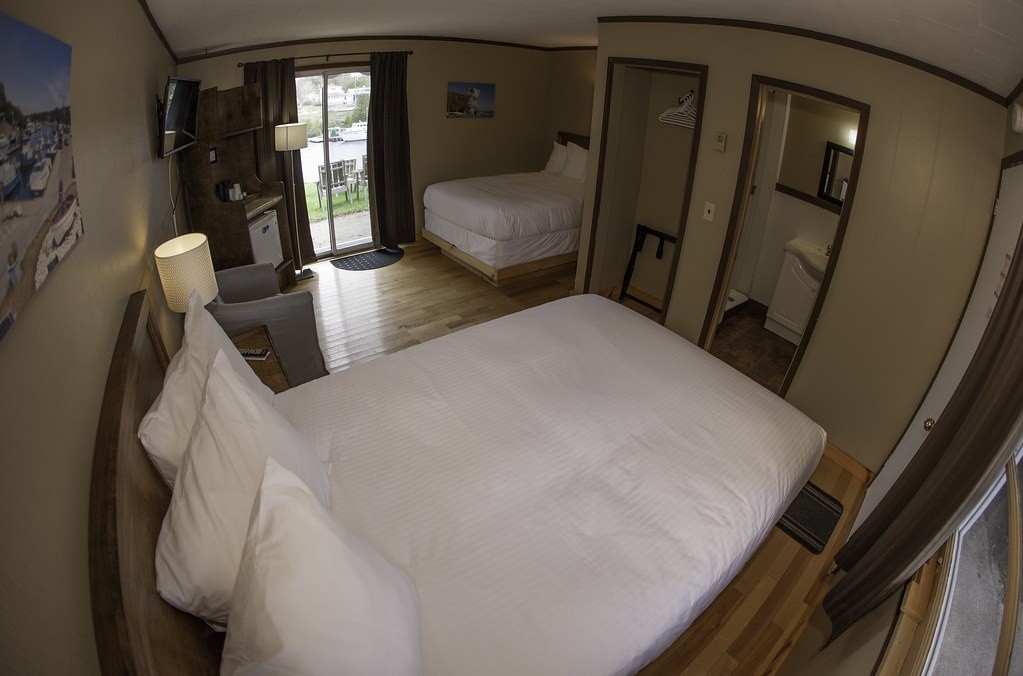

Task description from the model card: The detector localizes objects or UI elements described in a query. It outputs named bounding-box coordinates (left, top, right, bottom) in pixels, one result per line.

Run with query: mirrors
left=819, top=141, right=854, bottom=207
left=775, top=95, right=861, bottom=211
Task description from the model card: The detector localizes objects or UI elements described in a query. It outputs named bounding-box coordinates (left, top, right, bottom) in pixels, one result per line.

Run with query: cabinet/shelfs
left=185, top=83, right=298, bottom=292
left=763, top=249, right=820, bottom=345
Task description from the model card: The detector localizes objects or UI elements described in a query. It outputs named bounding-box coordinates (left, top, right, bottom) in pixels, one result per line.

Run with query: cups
left=216, top=181, right=246, bottom=202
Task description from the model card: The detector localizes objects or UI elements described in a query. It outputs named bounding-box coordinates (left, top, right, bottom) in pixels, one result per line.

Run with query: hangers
left=658, top=91, right=698, bottom=130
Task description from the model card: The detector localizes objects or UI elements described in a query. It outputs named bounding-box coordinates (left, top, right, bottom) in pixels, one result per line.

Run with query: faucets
left=825, top=245, right=832, bottom=256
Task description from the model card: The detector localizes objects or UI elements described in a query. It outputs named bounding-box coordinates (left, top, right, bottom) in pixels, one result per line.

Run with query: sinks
left=804, top=251, right=831, bottom=265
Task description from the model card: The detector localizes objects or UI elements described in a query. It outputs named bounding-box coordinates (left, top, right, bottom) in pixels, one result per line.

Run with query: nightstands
left=230, top=325, right=294, bottom=393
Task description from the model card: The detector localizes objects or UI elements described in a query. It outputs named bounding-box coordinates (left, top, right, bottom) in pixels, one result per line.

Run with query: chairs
left=316, top=154, right=368, bottom=209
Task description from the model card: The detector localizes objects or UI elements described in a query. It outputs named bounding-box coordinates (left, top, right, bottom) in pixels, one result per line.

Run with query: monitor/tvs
left=157, top=76, right=202, bottom=159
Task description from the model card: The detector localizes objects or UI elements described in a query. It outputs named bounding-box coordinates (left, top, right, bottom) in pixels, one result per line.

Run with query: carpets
left=329, top=246, right=404, bottom=272
left=775, top=479, right=845, bottom=555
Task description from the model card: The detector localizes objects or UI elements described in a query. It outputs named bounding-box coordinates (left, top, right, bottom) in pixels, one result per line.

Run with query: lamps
left=275, top=123, right=316, bottom=282
left=155, top=233, right=219, bottom=314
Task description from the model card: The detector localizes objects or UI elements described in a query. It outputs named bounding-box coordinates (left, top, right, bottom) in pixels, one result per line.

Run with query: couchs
left=207, top=263, right=329, bottom=393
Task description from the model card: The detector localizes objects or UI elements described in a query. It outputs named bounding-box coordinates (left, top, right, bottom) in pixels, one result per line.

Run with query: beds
left=422, top=132, right=590, bottom=287
left=91, top=293, right=827, bottom=676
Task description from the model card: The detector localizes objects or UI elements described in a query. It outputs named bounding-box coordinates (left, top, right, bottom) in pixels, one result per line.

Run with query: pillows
left=219, top=457, right=426, bottom=675
left=135, top=290, right=275, bottom=489
left=156, top=347, right=330, bottom=635
left=545, top=141, right=589, bottom=179
